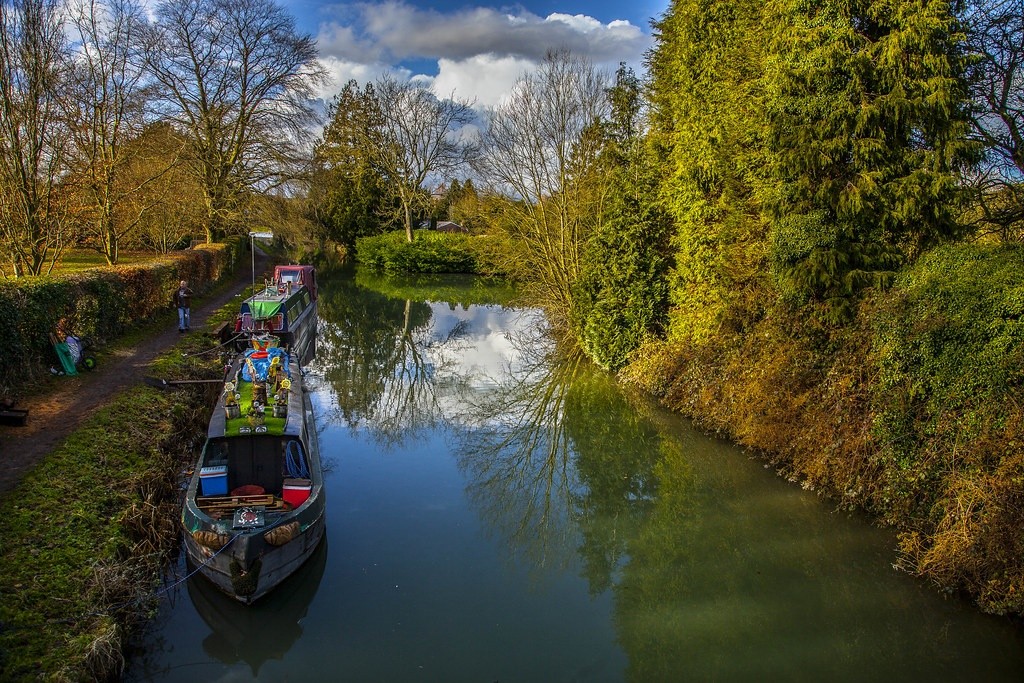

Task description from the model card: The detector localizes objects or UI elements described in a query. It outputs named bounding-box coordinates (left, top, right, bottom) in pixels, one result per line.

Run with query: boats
left=230, top=263, right=319, bottom=363
left=184, top=525, right=328, bottom=678
left=180, top=331, right=327, bottom=606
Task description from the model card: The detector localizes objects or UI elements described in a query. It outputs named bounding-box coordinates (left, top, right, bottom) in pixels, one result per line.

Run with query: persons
left=173, top=281, right=194, bottom=333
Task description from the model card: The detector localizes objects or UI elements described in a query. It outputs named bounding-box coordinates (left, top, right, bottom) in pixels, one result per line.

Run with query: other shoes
left=179, top=329, right=184, bottom=333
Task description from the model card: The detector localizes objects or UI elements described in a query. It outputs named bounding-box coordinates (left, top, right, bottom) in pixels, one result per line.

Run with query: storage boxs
left=282, top=478, right=312, bottom=510
left=200, top=465, right=230, bottom=497
